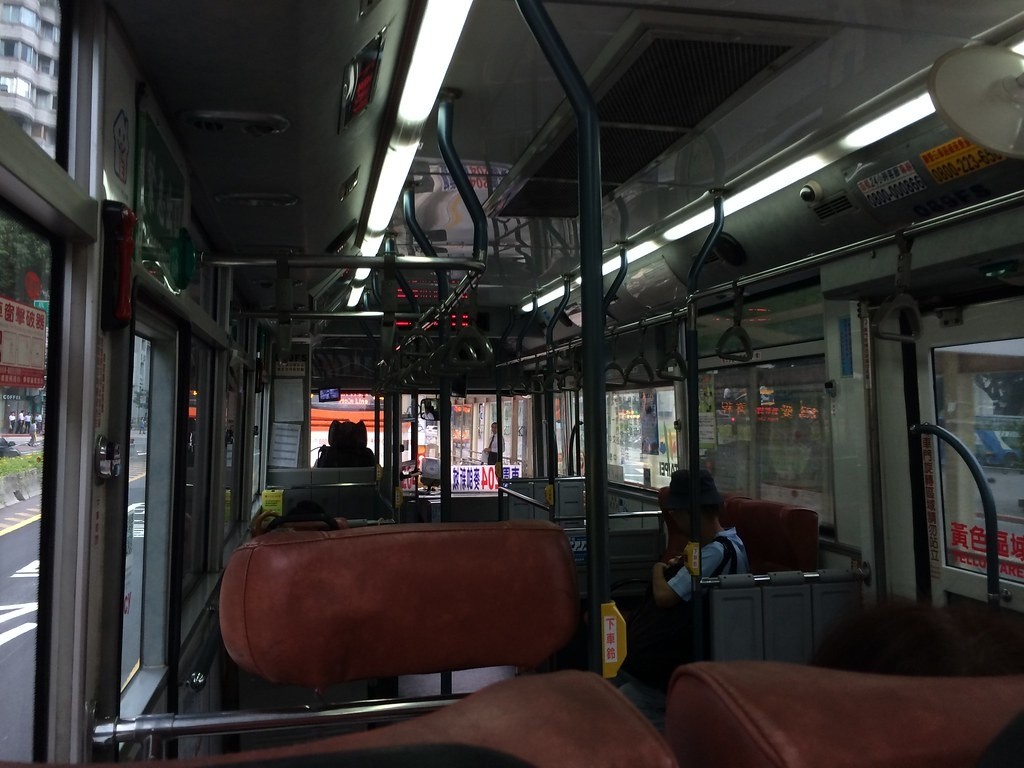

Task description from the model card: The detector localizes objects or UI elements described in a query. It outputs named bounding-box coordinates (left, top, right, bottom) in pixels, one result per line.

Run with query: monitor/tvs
left=318, top=385, right=341, bottom=402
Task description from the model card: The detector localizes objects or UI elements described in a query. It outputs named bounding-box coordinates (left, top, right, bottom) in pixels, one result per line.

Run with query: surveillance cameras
left=801, top=180, right=823, bottom=203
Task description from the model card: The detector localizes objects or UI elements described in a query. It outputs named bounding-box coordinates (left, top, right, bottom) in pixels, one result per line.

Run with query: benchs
left=0, top=466, right=1024, bottom=768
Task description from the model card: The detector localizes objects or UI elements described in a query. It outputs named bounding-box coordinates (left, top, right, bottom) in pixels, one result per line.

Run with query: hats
left=665, top=469, right=723, bottom=506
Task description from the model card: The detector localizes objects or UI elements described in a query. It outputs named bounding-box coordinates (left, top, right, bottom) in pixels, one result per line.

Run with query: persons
left=8, top=410, right=43, bottom=447
left=484, top=422, right=505, bottom=465
left=619, top=469, right=750, bottom=739
left=139, top=417, right=146, bottom=434
left=845, top=594, right=950, bottom=677
